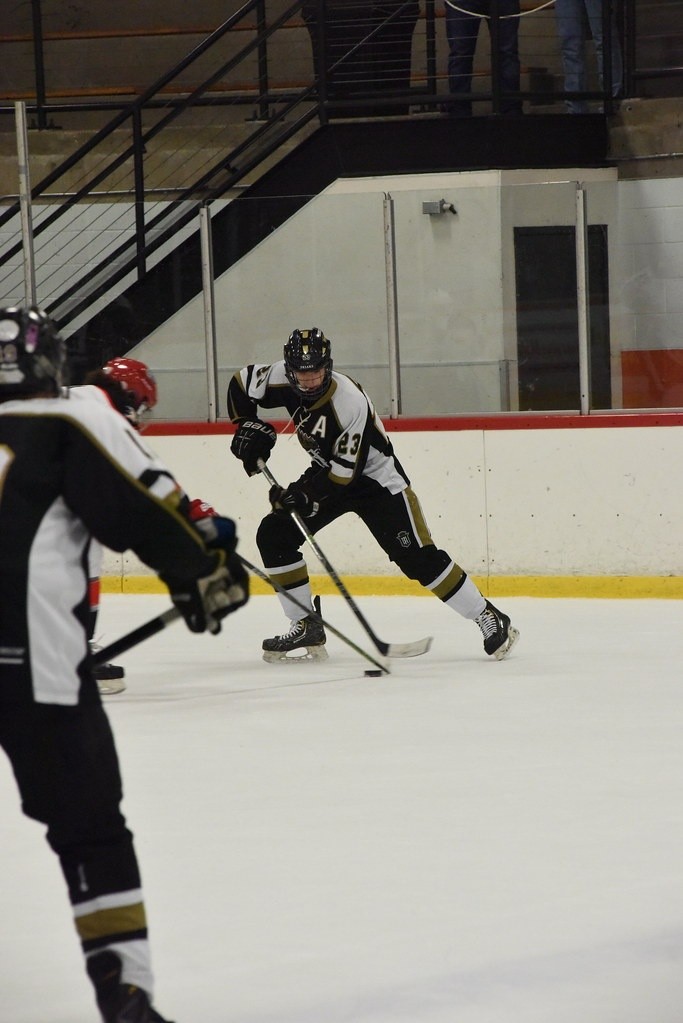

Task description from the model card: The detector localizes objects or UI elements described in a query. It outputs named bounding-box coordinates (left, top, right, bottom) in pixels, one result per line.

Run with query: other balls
left=364, top=669, right=382, bottom=677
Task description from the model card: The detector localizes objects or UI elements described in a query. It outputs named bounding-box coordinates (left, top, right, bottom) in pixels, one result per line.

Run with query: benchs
left=0, top=0, right=557, bottom=126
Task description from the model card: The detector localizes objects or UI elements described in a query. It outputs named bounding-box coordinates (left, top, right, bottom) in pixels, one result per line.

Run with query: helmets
left=283, top=327, right=334, bottom=403
left=104, top=357, right=158, bottom=413
left=0, top=305, right=68, bottom=395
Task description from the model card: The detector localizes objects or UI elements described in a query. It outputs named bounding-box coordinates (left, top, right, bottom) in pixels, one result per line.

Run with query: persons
left=554, top=0, right=629, bottom=114
left=1, top=308, right=250, bottom=1023
left=439, top=0, right=523, bottom=115
left=227, top=329, right=511, bottom=657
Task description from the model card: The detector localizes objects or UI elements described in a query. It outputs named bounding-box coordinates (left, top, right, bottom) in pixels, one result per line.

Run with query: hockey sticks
left=256, top=456, right=435, bottom=660
left=232, top=551, right=393, bottom=678
left=89, top=576, right=232, bottom=666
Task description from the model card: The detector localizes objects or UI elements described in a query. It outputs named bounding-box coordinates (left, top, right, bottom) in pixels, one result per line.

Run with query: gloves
left=268, top=470, right=321, bottom=521
left=163, top=517, right=250, bottom=636
left=229, top=419, right=277, bottom=477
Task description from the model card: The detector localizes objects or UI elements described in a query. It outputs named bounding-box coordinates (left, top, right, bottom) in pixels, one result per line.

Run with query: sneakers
left=262, top=594, right=330, bottom=662
left=90, top=643, right=126, bottom=696
left=474, top=598, right=519, bottom=661
